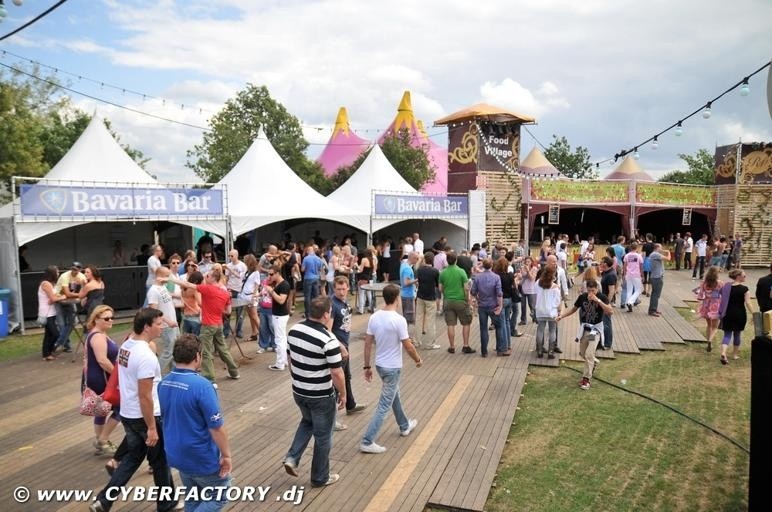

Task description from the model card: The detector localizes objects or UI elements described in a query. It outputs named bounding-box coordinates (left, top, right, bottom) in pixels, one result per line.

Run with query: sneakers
left=401, top=419, right=417, bottom=436
left=489, top=323, right=495, bottom=330
left=512, top=321, right=526, bottom=336
left=536, top=347, right=562, bottom=358
left=42, top=342, right=72, bottom=361
left=360, top=442, right=385, bottom=453
left=211, top=331, right=288, bottom=389
left=89, top=440, right=185, bottom=511
left=334, top=404, right=366, bottom=431
left=415, top=341, right=512, bottom=357
left=707, top=342, right=729, bottom=365
left=580, top=359, right=600, bottom=389
left=621, top=291, right=662, bottom=317
left=283, top=460, right=339, bottom=486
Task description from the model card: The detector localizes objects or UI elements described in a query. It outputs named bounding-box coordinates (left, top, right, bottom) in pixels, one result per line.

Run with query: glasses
left=99, top=317, right=114, bottom=321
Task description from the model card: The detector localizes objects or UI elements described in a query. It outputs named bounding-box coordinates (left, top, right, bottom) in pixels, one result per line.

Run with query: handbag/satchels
left=37, top=317, right=48, bottom=326
left=359, top=280, right=369, bottom=286
left=512, top=286, right=521, bottom=302
left=104, top=362, right=120, bottom=407
left=80, top=387, right=112, bottom=416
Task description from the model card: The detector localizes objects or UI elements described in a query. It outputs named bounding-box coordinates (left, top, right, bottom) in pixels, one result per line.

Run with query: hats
left=72, top=262, right=82, bottom=270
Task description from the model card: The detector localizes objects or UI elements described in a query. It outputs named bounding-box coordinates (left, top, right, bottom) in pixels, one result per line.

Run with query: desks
left=224, top=298, right=251, bottom=370
left=359, top=281, right=398, bottom=315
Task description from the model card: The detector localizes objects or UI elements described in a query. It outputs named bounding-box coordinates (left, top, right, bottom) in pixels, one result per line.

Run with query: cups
left=369, top=280, right=373, bottom=287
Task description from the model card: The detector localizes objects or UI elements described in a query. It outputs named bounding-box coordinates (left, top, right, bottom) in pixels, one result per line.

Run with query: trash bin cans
left=0, top=287, right=12, bottom=338
